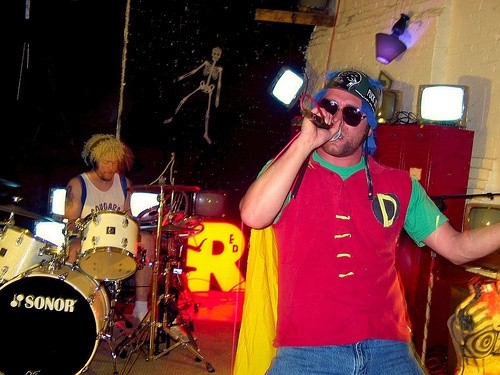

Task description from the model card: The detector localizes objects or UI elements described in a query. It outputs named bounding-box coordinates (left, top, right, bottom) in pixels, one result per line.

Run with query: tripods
left=119, top=188, right=215, bottom=375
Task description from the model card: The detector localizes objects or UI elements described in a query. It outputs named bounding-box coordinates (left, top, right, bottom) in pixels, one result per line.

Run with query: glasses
left=319, top=98, right=366, bottom=128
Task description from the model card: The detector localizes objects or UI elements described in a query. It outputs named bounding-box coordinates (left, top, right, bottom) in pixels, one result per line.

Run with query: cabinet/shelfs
left=291, top=118, right=478, bottom=352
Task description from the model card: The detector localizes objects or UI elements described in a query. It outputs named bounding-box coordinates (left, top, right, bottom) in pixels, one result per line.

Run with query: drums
left=77, top=209, right=140, bottom=281
left=0, top=273, right=104, bottom=374
left=114, top=229, right=157, bottom=303
left=0, top=225, right=58, bottom=284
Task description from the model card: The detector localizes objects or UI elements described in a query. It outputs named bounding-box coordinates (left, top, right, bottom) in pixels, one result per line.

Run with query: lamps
left=462, top=202, right=500, bottom=231
left=268, top=64, right=307, bottom=110
left=31, top=187, right=68, bottom=245
left=375, top=14, right=410, bottom=64
left=417, top=83, right=469, bottom=128
left=194, top=189, right=230, bottom=218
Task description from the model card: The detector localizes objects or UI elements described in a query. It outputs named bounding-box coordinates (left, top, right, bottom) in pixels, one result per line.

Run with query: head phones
left=84, top=137, right=116, bottom=166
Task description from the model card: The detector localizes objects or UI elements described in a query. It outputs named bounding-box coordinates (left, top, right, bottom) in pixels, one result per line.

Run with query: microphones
left=302, top=108, right=342, bottom=141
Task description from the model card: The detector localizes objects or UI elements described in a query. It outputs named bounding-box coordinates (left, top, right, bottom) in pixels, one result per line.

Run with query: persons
left=65, top=135, right=155, bottom=321
left=239, top=70, right=500, bottom=375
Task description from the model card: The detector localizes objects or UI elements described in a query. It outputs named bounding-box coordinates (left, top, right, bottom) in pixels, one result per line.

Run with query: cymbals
left=0, top=204, right=52, bottom=222
left=126, top=184, right=201, bottom=194
left=155, top=224, right=197, bottom=232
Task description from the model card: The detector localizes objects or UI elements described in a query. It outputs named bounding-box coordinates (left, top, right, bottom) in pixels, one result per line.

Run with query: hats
left=326, top=70, right=381, bottom=114
